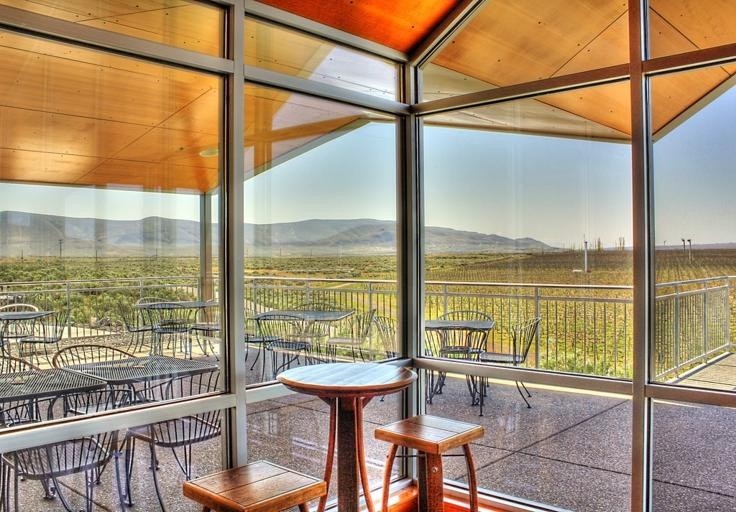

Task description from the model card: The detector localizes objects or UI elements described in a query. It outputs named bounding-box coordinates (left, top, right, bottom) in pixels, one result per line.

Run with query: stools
left=373, top=412, right=484, bottom=512
left=182, top=457, right=327, bottom=512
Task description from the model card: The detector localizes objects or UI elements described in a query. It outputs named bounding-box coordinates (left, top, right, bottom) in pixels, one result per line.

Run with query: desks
left=275, top=361, right=419, bottom=512
left=142, top=301, right=220, bottom=356
left=63, top=354, right=218, bottom=481
left=247, top=309, right=355, bottom=371
left=0, top=367, right=108, bottom=500
left=419, top=318, right=495, bottom=404
left=0, top=311, right=56, bottom=365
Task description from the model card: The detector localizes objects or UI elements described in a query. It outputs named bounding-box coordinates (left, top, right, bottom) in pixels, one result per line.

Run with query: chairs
left=372, top=315, right=433, bottom=406
left=139, top=297, right=177, bottom=353
left=117, top=302, right=156, bottom=358
left=190, top=304, right=222, bottom=361
left=244, top=308, right=280, bottom=371
left=325, top=308, right=376, bottom=364
left=478, top=317, right=543, bottom=417
left=1, top=389, right=132, bottom=511
left=435, top=310, right=495, bottom=406
left=51, top=343, right=143, bottom=486
left=0, top=303, right=41, bottom=369
left=256, top=313, right=313, bottom=380
left=119, top=368, right=223, bottom=507
left=291, top=301, right=337, bottom=365
left=19, top=308, right=70, bottom=372
left=147, top=303, right=192, bottom=361
left=0, top=355, right=56, bottom=480
